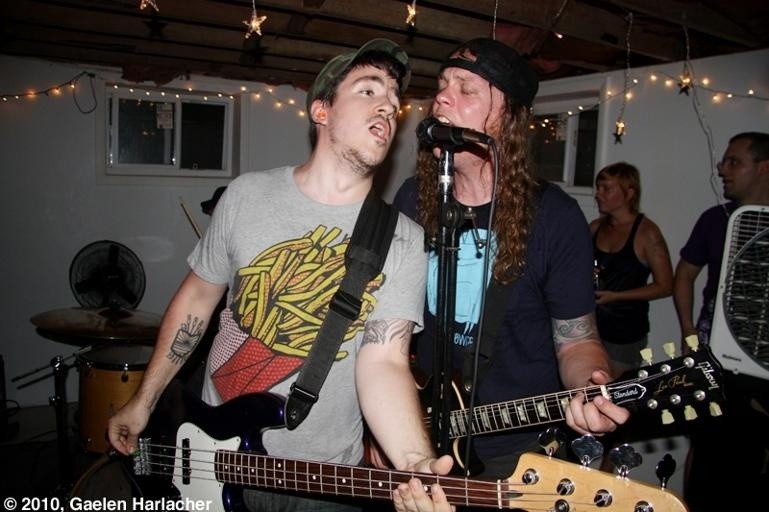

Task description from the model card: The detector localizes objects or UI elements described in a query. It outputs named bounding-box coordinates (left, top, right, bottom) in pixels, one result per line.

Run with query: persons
left=106, top=36, right=461, bottom=512
left=585, top=162, right=674, bottom=476
left=165, top=185, right=229, bottom=426
left=669, top=130, right=769, bottom=511
left=391, top=37, right=632, bottom=485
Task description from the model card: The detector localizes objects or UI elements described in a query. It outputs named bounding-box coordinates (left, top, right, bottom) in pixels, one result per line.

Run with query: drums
left=74, top=340, right=156, bottom=454
left=4, top=442, right=137, bottom=512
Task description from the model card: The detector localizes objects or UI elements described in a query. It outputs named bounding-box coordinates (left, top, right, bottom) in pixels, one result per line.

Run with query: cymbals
left=30, top=305, right=162, bottom=339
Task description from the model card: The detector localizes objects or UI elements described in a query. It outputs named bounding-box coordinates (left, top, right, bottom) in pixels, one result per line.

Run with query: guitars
left=122, top=332, right=724, bottom=512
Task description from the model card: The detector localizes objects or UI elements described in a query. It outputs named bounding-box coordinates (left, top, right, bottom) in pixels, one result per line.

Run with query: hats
left=438, top=37, right=539, bottom=107
left=306, top=37, right=412, bottom=116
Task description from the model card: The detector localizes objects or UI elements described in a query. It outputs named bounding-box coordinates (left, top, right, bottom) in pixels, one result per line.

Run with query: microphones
left=416, top=117, right=493, bottom=145
